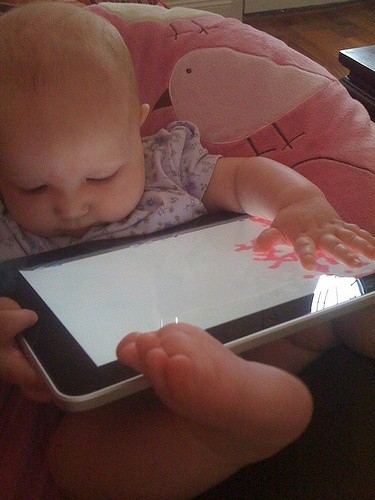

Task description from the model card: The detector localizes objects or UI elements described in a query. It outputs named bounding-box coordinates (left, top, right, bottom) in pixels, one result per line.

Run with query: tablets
left=1, top=211, right=375, bottom=410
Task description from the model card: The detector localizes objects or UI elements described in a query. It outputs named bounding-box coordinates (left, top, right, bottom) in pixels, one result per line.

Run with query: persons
left=0, top=1, right=375, bottom=500
